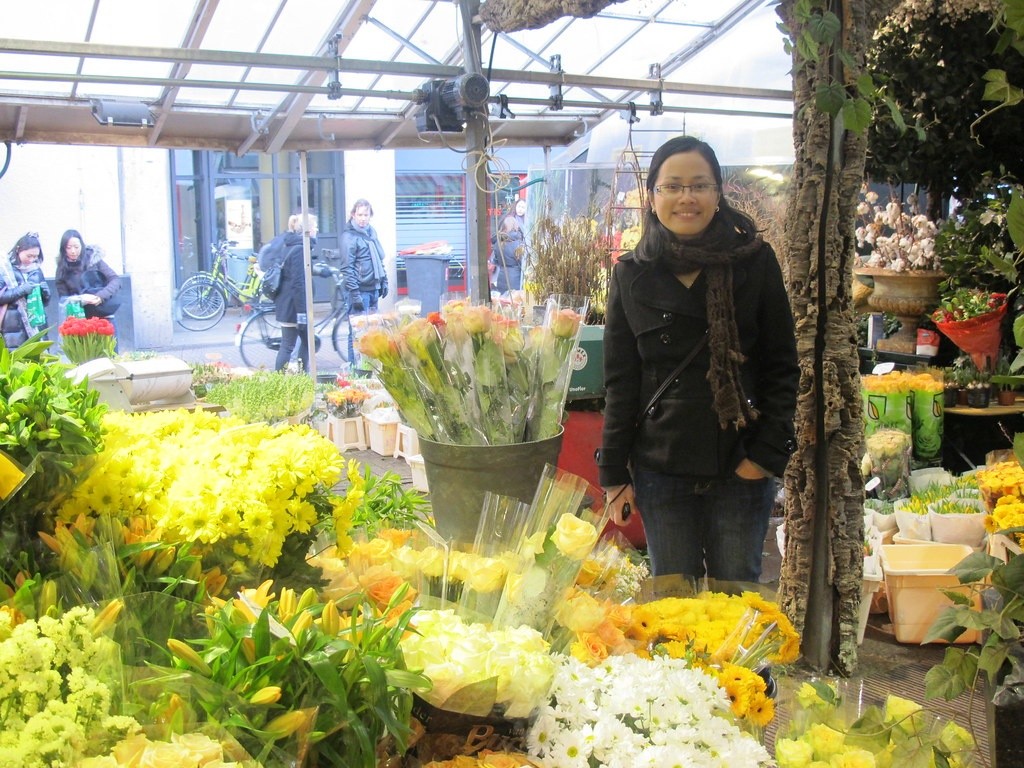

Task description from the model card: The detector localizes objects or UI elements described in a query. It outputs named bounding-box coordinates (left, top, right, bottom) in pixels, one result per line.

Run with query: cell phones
left=622, top=503, right=630, bottom=521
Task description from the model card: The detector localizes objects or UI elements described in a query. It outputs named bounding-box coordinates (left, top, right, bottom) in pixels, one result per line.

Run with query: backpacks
left=262, top=244, right=309, bottom=300
left=258, top=233, right=299, bottom=273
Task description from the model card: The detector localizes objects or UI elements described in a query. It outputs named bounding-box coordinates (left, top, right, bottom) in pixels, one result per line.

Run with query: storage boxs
left=892, top=531, right=987, bottom=552
left=856, top=563, right=884, bottom=646
left=408, top=454, right=430, bottom=493
left=880, top=544, right=984, bottom=644
left=365, top=406, right=402, bottom=457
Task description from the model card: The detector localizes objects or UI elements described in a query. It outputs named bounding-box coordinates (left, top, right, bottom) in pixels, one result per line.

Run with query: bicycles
left=174, top=236, right=316, bottom=332
left=235, top=261, right=379, bottom=373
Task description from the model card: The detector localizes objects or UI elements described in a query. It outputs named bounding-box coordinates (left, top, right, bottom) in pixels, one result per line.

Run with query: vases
left=394, top=423, right=421, bottom=464
left=326, top=413, right=368, bottom=452
left=853, top=268, right=948, bottom=354
left=417, top=422, right=565, bottom=554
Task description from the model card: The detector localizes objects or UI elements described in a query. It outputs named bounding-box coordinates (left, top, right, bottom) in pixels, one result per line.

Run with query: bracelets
left=607, top=483, right=629, bottom=507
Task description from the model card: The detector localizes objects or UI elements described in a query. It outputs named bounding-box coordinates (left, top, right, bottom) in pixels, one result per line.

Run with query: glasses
left=653, top=183, right=718, bottom=199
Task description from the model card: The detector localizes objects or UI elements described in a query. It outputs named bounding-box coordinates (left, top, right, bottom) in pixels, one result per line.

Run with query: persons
left=0, top=233, right=51, bottom=353
left=599, top=134, right=799, bottom=701
left=55, top=229, right=121, bottom=357
left=263, top=213, right=331, bottom=376
left=336, top=198, right=388, bottom=369
left=492, top=214, right=525, bottom=296
left=489, top=197, right=526, bottom=291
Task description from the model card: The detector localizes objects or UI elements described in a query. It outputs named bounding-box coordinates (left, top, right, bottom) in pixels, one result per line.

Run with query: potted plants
left=514, top=192, right=606, bottom=402
left=938, top=365, right=1016, bottom=408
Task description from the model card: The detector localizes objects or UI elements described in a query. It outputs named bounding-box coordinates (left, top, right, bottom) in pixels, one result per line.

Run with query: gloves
left=40, top=282, right=50, bottom=298
left=319, top=268, right=331, bottom=278
left=297, top=324, right=307, bottom=334
left=353, top=295, right=364, bottom=312
left=17, top=282, right=36, bottom=294
left=380, top=282, right=388, bottom=298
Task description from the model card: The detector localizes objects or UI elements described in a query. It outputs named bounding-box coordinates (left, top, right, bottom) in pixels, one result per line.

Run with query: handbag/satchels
left=81, top=273, right=120, bottom=316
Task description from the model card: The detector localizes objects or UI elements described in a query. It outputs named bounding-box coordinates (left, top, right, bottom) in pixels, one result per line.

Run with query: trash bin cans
left=399, top=253, right=455, bottom=318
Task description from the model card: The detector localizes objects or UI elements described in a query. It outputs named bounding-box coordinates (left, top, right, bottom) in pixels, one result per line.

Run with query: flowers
left=855, top=171, right=939, bottom=271
left=57, top=315, right=115, bottom=367
left=924, top=286, right=1009, bottom=373
left=320, top=370, right=371, bottom=418
left=353, top=293, right=592, bottom=446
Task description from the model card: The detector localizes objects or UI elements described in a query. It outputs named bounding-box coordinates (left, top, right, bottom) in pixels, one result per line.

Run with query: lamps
left=89, top=96, right=155, bottom=127
